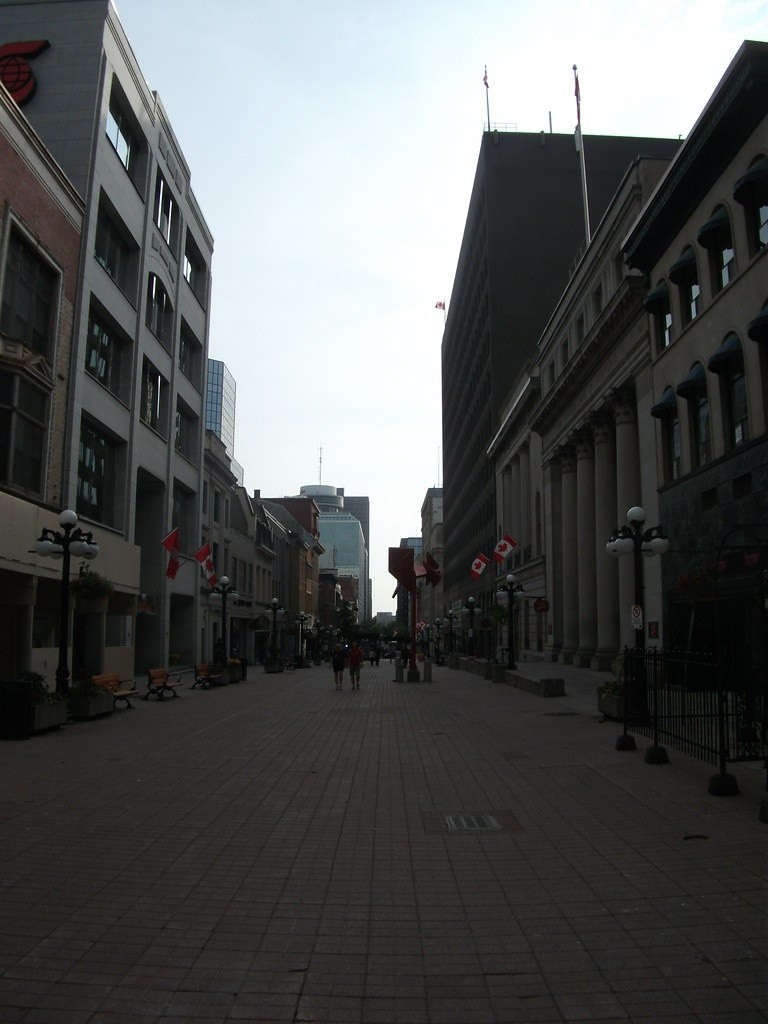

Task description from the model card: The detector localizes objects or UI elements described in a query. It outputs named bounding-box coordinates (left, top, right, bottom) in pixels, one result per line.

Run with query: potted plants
left=215, top=666, right=230, bottom=685
left=69, top=680, right=113, bottom=717
left=598, top=681, right=625, bottom=720
left=22, top=671, right=66, bottom=731
left=312, top=655, right=321, bottom=666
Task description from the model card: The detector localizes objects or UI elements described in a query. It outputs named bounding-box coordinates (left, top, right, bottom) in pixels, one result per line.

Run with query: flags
left=195, top=545, right=217, bottom=585
left=469, top=553, right=490, bottom=580
left=162, top=528, right=181, bottom=579
left=492, top=535, right=517, bottom=563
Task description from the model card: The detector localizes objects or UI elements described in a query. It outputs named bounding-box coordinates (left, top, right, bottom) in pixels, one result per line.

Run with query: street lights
left=294, top=611, right=313, bottom=655
left=325, top=625, right=341, bottom=655
left=605, top=506, right=672, bottom=725
left=423, top=624, right=434, bottom=658
left=264, top=597, right=285, bottom=660
left=311, top=619, right=325, bottom=653
left=35, top=510, right=101, bottom=689
left=496, top=574, right=526, bottom=669
left=460, top=596, right=483, bottom=656
left=443, top=609, right=459, bottom=656
left=432, top=617, right=444, bottom=659
left=207, top=575, right=240, bottom=657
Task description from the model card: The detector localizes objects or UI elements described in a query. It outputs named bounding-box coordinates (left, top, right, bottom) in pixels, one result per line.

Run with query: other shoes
left=336, top=687, right=339, bottom=691
left=340, top=685, right=342, bottom=689
left=356, top=683, right=360, bottom=689
left=352, top=685, right=355, bottom=690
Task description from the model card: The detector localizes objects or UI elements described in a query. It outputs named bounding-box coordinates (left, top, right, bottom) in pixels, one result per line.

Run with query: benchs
left=280, top=655, right=296, bottom=670
left=142, top=668, right=184, bottom=700
left=190, top=663, right=223, bottom=690
left=90, top=672, right=140, bottom=709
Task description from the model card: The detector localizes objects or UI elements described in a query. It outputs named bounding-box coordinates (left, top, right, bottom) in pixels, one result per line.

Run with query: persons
left=346, top=642, right=362, bottom=689
left=328, top=643, right=346, bottom=691
left=401, top=646, right=410, bottom=668
left=369, top=648, right=379, bottom=666
